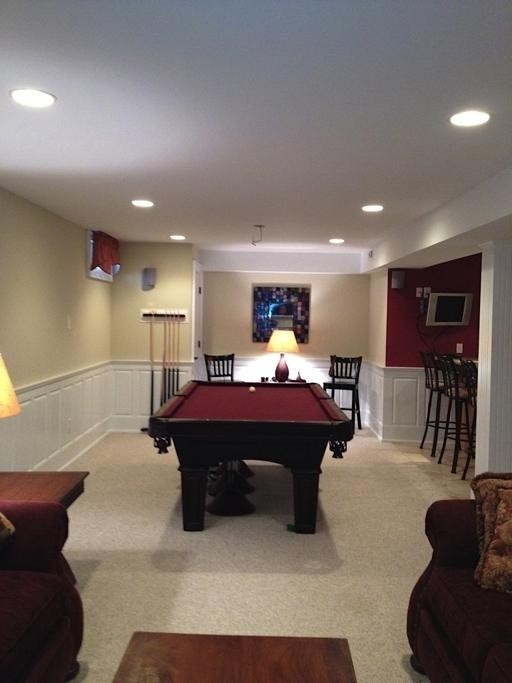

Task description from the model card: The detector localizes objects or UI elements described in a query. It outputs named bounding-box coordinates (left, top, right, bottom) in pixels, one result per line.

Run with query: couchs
left=403, top=499, right=512, bottom=683
left=0, top=500, right=83, bottom=683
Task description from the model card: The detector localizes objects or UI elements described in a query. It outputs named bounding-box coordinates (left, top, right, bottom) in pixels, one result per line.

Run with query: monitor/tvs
left=426, top=293, right=474, bottom=325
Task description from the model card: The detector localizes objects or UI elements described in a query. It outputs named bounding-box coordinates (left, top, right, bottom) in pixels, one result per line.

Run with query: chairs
left=437, top=352, right=476, bottom=474
left=324, top=355, right=363, bottom=435
left=461, top=358, right=479, bottom=480
left=420, top=349, right=462, bottom=456
left=204, top=352, right=235, bottom=381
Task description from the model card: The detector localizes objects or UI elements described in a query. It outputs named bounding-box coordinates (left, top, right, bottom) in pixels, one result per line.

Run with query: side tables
left=0, top=469, right=90, bottom=585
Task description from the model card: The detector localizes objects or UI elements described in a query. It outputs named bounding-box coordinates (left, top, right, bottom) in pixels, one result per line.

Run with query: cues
left=176, top=312, right=179, bottom=391
left=168, top=312, right=171, bottom=400
left=151, top=309, right=154, bottom=415
left=172, top=312, right=175, bottom=397
left=164, top=311, right=167, bottom=404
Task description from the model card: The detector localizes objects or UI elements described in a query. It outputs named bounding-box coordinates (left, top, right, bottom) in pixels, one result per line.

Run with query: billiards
left=249, top=387, right=256, bottom=392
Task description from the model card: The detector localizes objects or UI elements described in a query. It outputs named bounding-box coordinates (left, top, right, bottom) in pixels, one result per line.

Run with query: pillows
left=470, top=471, right=512, bottom=556
left=474, top=483, right=511, bottom=594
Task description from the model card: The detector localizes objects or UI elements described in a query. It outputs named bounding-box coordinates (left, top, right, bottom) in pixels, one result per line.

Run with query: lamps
left=0, top=349, right=21, bottom=422
left=264, top=329, right=301, bottom=382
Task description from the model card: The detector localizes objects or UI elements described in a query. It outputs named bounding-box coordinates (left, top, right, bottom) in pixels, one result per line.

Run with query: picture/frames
left=250, top=282, right=311, bottom=345
